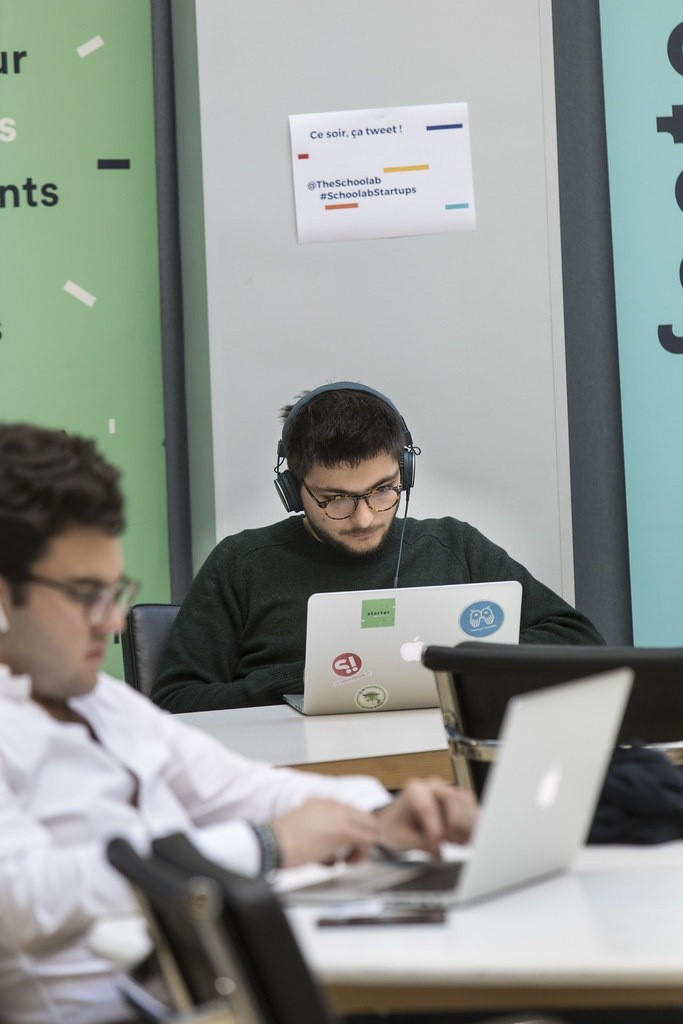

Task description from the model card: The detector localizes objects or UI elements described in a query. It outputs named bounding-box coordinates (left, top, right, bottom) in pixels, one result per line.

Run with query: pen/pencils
left=315, top=911, right=444, bottom=926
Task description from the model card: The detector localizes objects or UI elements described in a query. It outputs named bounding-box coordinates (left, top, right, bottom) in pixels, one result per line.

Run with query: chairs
left=424, top=639, right=683, bottom=806
left=110, top=830, right=334, bottom=1024
left=121, top=604, right=180, bottom=704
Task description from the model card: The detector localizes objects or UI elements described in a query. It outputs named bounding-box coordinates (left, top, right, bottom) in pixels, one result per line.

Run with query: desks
left=271, top=841, right=683, bottom=1019
left=184, top=704, right=456, bottom=790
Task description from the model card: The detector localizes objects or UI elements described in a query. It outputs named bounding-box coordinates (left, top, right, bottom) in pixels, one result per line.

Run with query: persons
left=0, top=422, right=478, bottom=1024
left=148, top=380, right=610, bottom=713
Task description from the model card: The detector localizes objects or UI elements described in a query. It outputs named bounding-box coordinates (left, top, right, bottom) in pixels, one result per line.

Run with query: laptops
left=275, top=665, right=635, bottom=910
left=282, top=580, right=522, bottom=716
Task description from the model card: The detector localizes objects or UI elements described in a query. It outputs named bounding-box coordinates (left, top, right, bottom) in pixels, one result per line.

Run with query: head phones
left=272, top=382, right=415, bottom=513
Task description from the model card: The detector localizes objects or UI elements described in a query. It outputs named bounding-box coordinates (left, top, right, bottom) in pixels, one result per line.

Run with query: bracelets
left=252, top=823, right=281, bottom=883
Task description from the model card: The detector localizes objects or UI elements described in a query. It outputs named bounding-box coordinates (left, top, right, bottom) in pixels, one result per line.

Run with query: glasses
left=301, top=478, right=404, bottom=520
left=26, top=574, right=140, bottom=627
